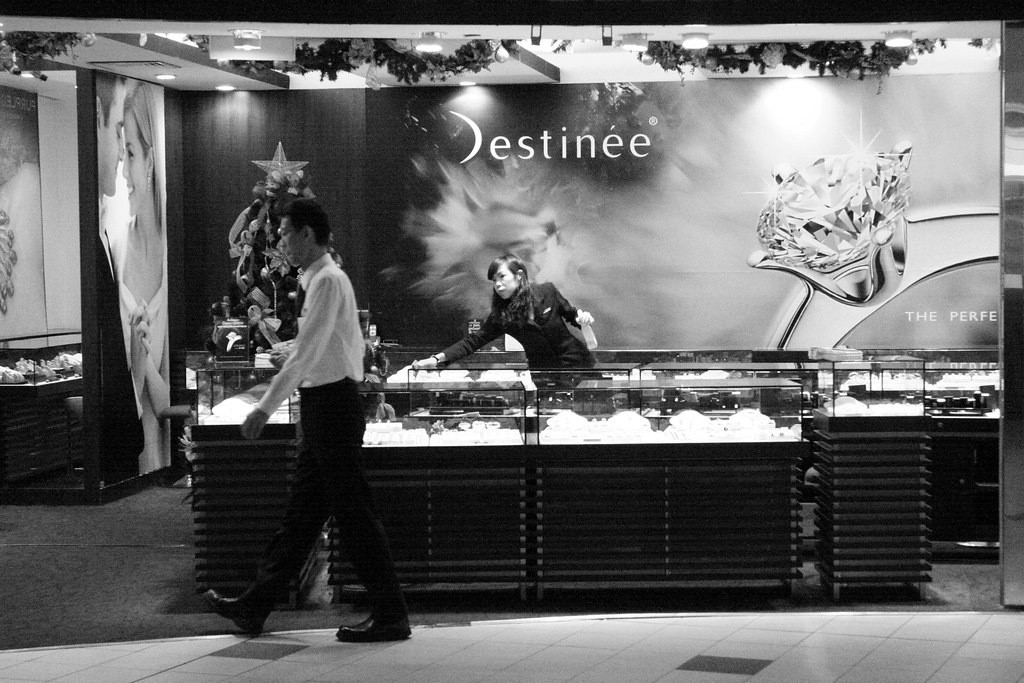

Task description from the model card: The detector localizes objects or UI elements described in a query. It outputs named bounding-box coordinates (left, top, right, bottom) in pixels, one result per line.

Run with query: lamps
left=416, top=31, right=444, bottom=53
left=681, top=33, right=709, bottom=50
left=618, top=33, right=648, bottom=53
left=79, top=32, right=98, bottom=47
left=229, top=30, right=263, bottom=51
left=884, top=30, right=913, bottom=48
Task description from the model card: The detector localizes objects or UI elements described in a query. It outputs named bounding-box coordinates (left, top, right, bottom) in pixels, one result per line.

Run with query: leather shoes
left=337, top=617, right=411, bottom=642
left=205, top=589, right=266, bottom=637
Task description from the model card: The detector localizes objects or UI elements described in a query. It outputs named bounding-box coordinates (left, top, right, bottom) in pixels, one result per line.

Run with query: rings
left=745, top=136, right=1000, bottom=350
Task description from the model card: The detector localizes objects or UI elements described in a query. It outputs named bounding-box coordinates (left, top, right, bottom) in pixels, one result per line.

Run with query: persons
left=412, top=253, right=596, bottom=414
left=202, top=197, right=412, bottom=643
left=90, top=69, right=168, bottom=491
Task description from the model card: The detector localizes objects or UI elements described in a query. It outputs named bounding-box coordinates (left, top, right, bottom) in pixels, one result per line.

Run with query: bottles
left=577, top=309, right=598, bottom=350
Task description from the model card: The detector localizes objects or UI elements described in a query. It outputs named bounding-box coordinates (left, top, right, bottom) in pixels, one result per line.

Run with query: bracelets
left=430, top=354, right=441, bottom=369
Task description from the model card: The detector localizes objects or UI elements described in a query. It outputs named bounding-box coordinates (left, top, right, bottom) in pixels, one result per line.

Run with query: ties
left=296, top=281, right=304, bottom=320
